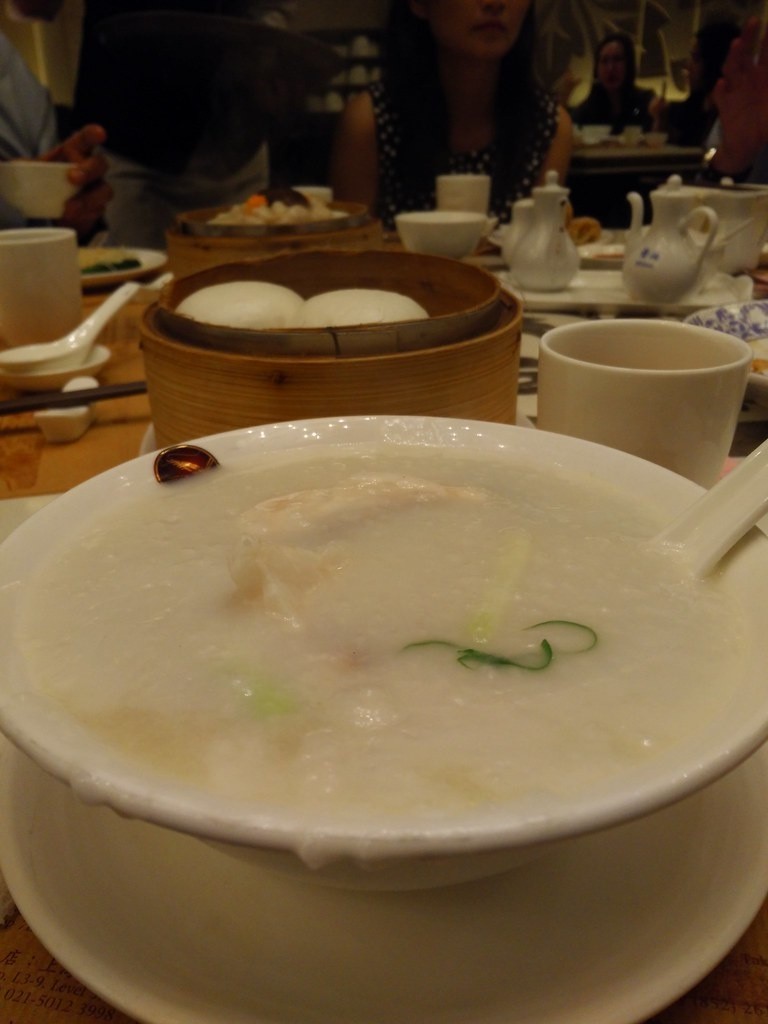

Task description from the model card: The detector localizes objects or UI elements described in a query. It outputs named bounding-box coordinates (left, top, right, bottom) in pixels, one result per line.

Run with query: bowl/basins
left=684, top=298, right=768, bottom=412
left=0, top=160, right=85, bottom=218
left=395, top=210, right=487, bottom=262
left=1, top=415, right=768, bottom=894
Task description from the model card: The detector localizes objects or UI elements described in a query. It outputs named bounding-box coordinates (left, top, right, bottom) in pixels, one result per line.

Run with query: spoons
left=0, top=282, right=141, bottom=371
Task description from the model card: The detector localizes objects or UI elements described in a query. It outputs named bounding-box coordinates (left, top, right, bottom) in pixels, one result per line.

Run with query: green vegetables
left=80, top=258, right=141, bottom=274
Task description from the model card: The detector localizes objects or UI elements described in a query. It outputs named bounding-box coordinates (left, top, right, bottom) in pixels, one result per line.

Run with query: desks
left=0, top=232, right=768, bottom=1024
left=565, top=145, right=706, bottom=231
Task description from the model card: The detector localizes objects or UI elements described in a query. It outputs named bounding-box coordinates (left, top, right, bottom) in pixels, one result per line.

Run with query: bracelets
left=700, top=162, right=753, bottom=184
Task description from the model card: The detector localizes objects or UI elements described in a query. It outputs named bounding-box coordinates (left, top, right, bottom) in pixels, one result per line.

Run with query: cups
left=0, top=228, right=83, bottom=349
left=570, top=123, right=667, bottom=150
left=702, top=182, right=768, bottom=275
left=539, top=318, right=753, bottom=488
left=437, top=175, right=491, bottom=215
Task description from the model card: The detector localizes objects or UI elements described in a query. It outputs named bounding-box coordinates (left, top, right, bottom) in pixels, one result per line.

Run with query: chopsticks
left=0, top=377, right=146, bottom=414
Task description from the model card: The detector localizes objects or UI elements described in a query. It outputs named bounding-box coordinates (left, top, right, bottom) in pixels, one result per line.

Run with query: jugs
left=509, top=171, right=576, bottom=292
left=622, top=174, right=716, bottom=304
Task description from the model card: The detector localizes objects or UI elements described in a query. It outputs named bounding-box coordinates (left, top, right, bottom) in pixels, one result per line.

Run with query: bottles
left=501, top=199, right=534, bottom=269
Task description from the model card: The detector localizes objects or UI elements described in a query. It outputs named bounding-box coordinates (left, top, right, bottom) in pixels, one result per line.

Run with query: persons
left=0, top=0, right=296, bottom=250
left=695, top=15, right=768, bottom=188
left=328, top=0, right=572, bottom=232
left=579, top=22, right=741, bottom=143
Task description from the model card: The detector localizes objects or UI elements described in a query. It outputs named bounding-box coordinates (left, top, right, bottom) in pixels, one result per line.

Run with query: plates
left=488, top=224, right=614, bottom=250
left=1, top=731, right=767, bottom=1024
left=0, top=343, right=111, bottom=392
left=80, top=247, right=168, bottom=286
left=575, top=244, right=628, bottom=264
left=495, top=270, right=754, bottom=315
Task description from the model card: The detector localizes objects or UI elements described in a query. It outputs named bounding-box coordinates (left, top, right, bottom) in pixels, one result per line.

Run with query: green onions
left=400, top=619, right=599, bottom=671
